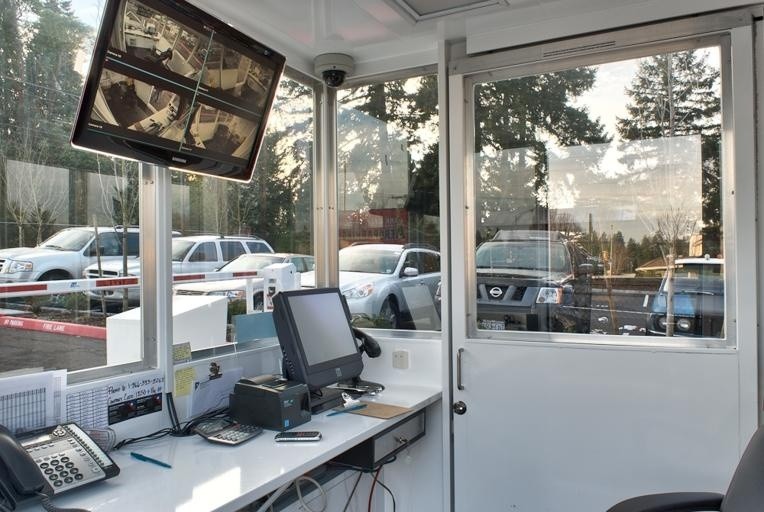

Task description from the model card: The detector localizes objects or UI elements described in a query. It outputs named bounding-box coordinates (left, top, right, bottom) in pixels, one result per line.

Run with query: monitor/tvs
left=69, top=0, right=288, bottom=185
left=271, top=286, right=364, bottom=414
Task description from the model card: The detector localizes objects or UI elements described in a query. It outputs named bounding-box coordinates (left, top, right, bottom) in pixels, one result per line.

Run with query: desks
left=15, top=383, right=442, bottom=512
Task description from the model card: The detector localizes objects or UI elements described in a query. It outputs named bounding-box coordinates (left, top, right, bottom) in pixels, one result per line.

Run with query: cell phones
left=274, top=431, right=323, bottom=442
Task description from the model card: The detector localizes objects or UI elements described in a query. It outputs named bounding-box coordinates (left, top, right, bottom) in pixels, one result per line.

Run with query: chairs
left=607, top=425, right=764, bottom=512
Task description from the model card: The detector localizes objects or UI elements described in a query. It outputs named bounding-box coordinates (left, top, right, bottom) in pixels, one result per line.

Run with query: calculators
left=191, top=418, right=263, bottom=445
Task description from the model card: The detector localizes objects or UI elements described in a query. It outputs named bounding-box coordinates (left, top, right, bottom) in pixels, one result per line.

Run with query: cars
left=298, top=241, right=441, bottom=329
left=171, top=251, right=314, bottom=307
left=0, top=223, right=179, bottom=284
left=82, top=232, right=274, bottom=308
left=644, top=254, right=724, bottom=340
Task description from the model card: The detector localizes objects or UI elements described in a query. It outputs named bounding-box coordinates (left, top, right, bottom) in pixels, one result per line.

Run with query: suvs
left=432, top=229, right=595, bottom=333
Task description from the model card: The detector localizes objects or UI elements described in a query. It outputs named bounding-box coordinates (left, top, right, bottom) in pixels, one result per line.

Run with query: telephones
left=0, top=421, right=122, bottom=511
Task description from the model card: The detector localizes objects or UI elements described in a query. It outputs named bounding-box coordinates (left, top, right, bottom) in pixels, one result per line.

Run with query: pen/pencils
left=326, top=403, right=368, bottom=417
left=130, top=452, right=172, bottom=468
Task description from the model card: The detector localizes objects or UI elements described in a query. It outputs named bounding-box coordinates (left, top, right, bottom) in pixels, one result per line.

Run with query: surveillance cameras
left=313, top=52, right=353, bottom=88
